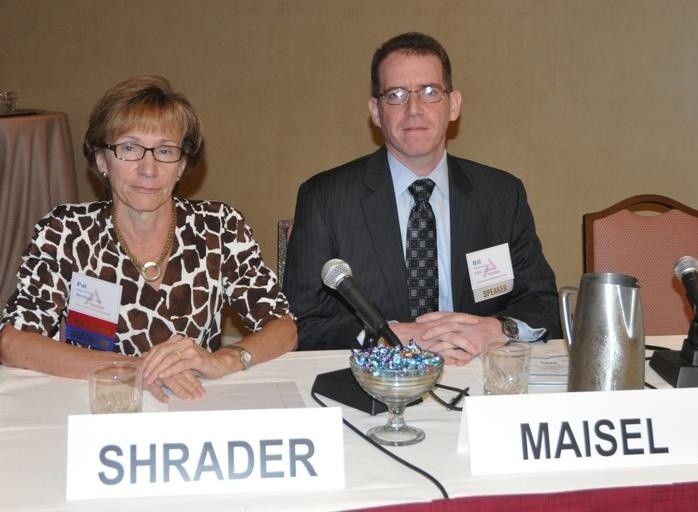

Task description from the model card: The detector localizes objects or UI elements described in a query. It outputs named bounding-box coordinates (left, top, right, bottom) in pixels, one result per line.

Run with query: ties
left=404, top=178, right=440, bottom=322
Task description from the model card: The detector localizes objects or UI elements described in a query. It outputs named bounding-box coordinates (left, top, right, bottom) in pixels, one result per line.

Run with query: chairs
left=582, top=193, right=697, bottom=335
left=274, top=213, right=295, bottom=288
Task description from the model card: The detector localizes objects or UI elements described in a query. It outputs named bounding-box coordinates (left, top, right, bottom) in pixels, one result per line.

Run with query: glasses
left=102, top=141, right=186, bottom=164
left=375, top=83, right=448, bottom=106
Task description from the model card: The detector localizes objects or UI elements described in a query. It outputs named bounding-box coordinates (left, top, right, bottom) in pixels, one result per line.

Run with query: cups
left=88, top=360, right=146, bottom=415
left=482, top=336, right=530, bottom=396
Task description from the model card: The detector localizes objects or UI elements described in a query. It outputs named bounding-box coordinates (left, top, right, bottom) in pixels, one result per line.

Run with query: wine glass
left=348, top=350, right=447, bottom=446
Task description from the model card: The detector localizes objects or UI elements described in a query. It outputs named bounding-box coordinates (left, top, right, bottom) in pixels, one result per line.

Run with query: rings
left=176, top=351, right=183, bottom=360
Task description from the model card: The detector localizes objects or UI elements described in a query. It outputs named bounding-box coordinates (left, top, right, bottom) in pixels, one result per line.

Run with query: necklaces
left=109, top=202, right=177, bottom=283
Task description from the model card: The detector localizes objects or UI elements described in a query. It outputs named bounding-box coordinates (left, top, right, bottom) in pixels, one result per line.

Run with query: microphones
left=672, top=255, right=698, bottom=325
left=320, top=258, right=404, bottom=350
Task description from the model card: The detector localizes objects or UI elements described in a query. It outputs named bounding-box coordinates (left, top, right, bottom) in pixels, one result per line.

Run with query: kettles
left=556, top=269, right=645, bottom=392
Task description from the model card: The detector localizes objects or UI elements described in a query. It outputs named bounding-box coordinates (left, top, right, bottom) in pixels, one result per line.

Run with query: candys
left=352, top=342, right=442, bottom=376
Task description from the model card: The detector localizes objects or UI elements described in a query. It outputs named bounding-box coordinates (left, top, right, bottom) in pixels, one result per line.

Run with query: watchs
left=220, top=344, right=253, bottom=372
left=493, top=314, right=520, bottom=347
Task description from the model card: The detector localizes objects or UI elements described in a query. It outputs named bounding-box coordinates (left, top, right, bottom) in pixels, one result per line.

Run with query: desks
left=0, top=338, right=697, bottom=510
left=2, top=110, right=77, bottom=315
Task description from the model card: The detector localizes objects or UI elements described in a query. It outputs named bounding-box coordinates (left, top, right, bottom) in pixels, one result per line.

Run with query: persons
left=0, top=74, right=301, bottom=404
left=281, top=32, right=566, bottom=369
